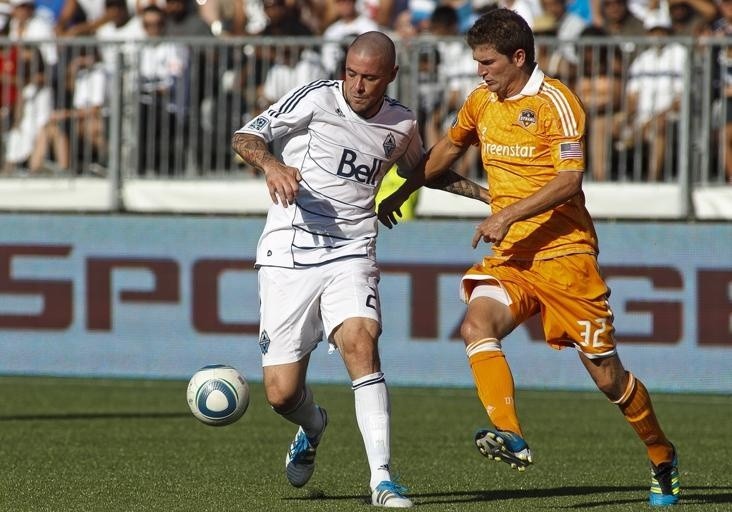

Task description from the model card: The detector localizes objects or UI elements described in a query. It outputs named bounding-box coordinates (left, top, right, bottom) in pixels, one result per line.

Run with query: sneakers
left=651, top=439, right=680, bottom=509
left=474, top=430, right=533, bottom=472
left=286, top=406, right=327, bottom=488
left=367, top=480, right=412, bottom=509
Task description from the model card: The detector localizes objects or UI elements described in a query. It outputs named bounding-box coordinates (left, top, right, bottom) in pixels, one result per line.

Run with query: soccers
left=187, top=363, right=251, bottom=427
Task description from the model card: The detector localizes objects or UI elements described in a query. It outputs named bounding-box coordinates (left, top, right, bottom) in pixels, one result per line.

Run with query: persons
left=0, top=0, right=732, bottom=182
left=377, top=8, right=681, bottom=507
left=233, top=32, right=494, bottom=507
